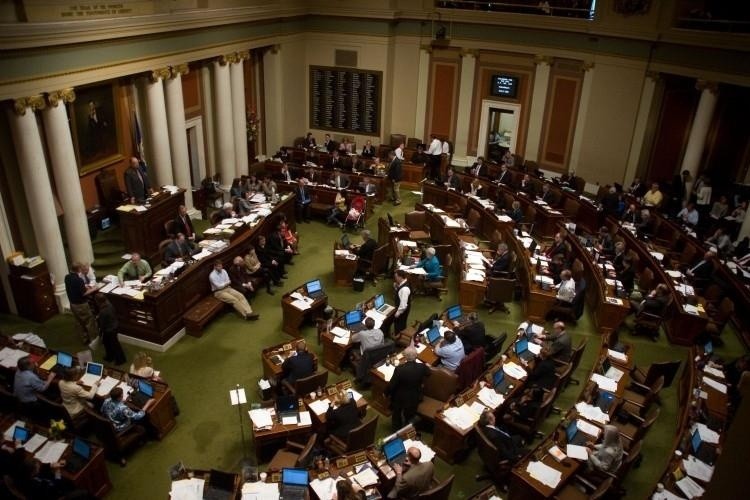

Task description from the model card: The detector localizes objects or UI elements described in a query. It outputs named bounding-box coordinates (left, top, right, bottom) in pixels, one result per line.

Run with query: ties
left=134, top=265, right=140, bottom=278
left=137, top=168, right=145, bottom=186
left=184, top=218, right=191, bottom=235
left=84, top=272, right=90, bottom=285
left=300, top=188, right=305, bottom=202
left=738, top=256, right=749, bottom=262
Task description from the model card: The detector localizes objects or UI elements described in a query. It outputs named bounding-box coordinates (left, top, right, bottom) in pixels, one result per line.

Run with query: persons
left=348, top=231, right=439, bottom=374
left=282, top=337, right=436, bottom=499
left=124, top=157, right=153, bottom=205
left=466, top=130, right=749, bottom=334
left=707, top=355, right=748, bottom=393
left=409, top=134, right=461, bottom=190
left=387, top=320, right=623, bottom=484
left=64, top=254, right=152, bottom=365
left=14, top=352, right=163, bottom=440
left=158, top=170, right=300, bottom=320
left=267, top=132, right=405, bottom=230
left=80, top=101, right=114, bottom=154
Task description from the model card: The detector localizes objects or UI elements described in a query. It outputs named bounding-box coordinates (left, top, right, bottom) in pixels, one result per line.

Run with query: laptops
left=374, top=294, right=395, bottom=316
left=536, top=257, right=552, bottom=275
left=525, top=321, right=539, bottom=344
left=519, top=223, right=532, bottom=237
left=48, top=351, right=73, bottom=378
left=699, top=398, right=723, bottom=433
left=593, top=249, right=609, bottom=264
left=387, top=212, right=400, bottom=226
left=447, top=304, right=468, bottom=327
left=127, top=379, right=155, bottom=409
left=204, top=468, right=237, bottom=500
left=609, top=329, right=630, bottom=353
left=275, top=395, right=301, bottom=422
left=344, top=309, right=367, bottom=332
left=601, top=357, right=625, bottom=382
left=602, top=262, right=617, bottom=278
left=515, top=337, right=536, bottom=364
left=381, top=436, right=408, bottom=474
left=587, top=383, right=613, bottom=415
left=690, top=427, right=718, bottom=466
left=12, top=424, right=29, bottom=448
left=426, top=324, right=445, bottom=347
left=492, top=368, right=515, bottom=395
left=704, top=339, right=722, bottom=364
left=77, top=362, right=103, bottom=387
left=279, top=467, right=309, bottom=500
left=541, top=274, right=553, bottom=292
left=614, top=278, right=630, bottom=299
left=305, top=278, right=325, bottom=299
left=564, top=418, right=587, bottom=448
left=528, top=239, right=540, bottom=255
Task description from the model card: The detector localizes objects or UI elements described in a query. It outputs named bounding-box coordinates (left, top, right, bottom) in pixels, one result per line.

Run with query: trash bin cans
left=353, top=279, right=365, bottom=292
left=258, top=377, right=272, bottom=401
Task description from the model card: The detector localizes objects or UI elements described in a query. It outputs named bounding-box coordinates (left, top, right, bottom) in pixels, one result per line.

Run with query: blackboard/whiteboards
left=308, top=63, right=383, bottom=138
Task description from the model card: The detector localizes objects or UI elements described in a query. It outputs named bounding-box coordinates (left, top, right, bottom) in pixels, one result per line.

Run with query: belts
left=212, top=286, right=227, bottom=291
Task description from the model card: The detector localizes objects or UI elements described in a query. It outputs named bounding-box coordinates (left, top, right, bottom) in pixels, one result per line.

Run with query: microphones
left=144, top=194, right=153, bottom=197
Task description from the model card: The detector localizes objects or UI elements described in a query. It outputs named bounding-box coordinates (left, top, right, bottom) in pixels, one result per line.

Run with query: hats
left=426, top=247, right=435, bottom=255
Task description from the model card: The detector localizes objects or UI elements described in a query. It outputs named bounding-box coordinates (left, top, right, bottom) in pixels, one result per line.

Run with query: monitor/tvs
left=489, top=72, right=519, bottom=98
